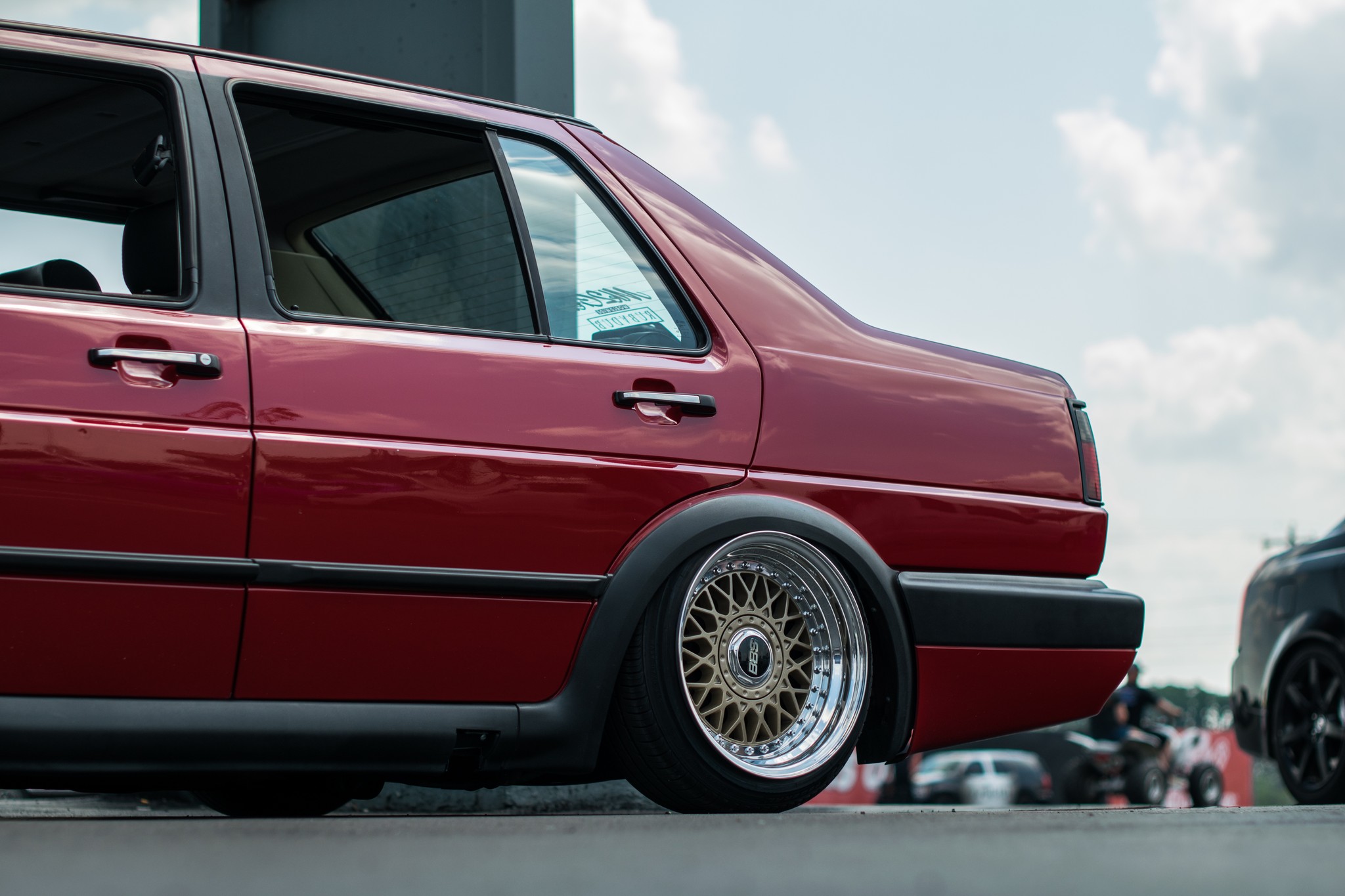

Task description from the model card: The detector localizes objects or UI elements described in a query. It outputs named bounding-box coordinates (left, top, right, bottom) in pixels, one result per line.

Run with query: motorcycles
left=1126, top=707, right=1223, bottom=810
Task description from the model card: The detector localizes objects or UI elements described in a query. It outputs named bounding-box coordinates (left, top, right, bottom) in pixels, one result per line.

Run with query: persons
left=1089, top=662, right=1182, bottom=773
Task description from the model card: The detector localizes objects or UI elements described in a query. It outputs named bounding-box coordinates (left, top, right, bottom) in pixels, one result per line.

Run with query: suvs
left=908, top=729, right=1124, bottom=806
left=0, top=16, right=1146, bottom=813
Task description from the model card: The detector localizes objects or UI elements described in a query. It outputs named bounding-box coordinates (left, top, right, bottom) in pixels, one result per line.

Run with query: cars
left=1230, top=520, right=1345, bottom=806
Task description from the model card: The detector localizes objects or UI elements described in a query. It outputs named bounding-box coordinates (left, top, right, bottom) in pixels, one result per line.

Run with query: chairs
left=0, top=259, right=102, bottom=292
left=122, top=198, right=178, bottom=297
